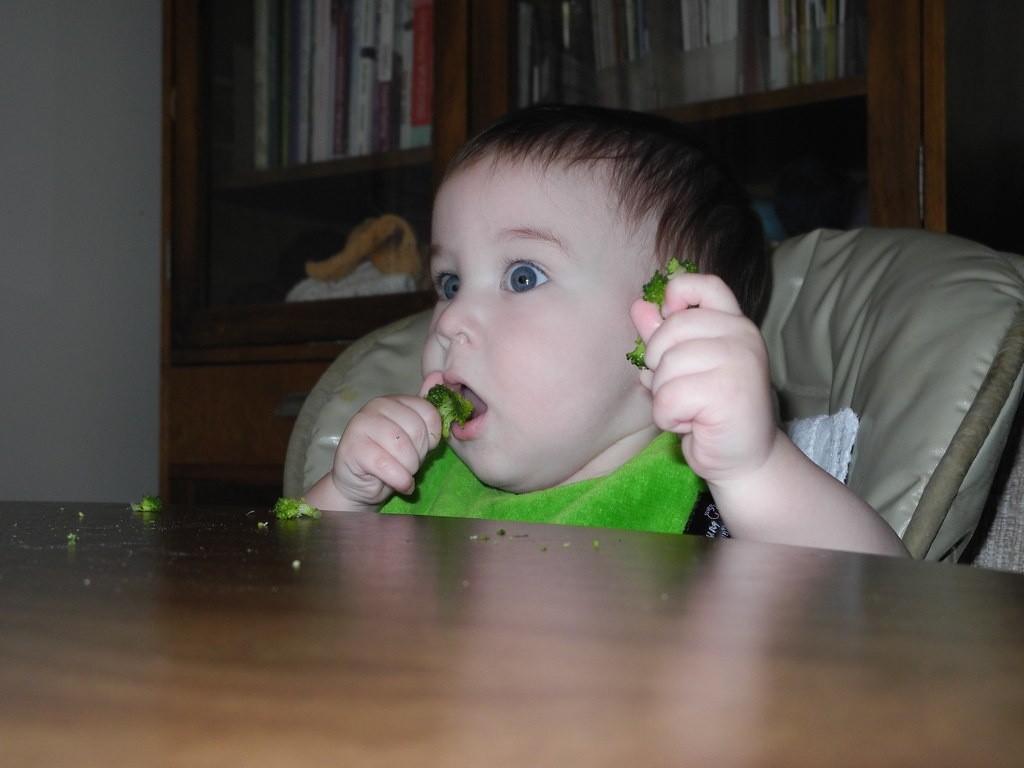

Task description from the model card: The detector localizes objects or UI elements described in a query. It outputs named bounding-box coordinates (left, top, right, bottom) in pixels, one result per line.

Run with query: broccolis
left=422, top=383, right=474, bottom=437
left=273, top=495, right=324, bottom=519
left=625, top=257, right=702, bottom=371
left=130, top=494, right=165, bottom=512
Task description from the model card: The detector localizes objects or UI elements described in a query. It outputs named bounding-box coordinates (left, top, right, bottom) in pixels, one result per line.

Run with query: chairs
left=282, top=228, right=1023, bottom=581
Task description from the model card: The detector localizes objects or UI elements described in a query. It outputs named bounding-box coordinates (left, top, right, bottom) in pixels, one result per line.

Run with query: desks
left=0, top=494, right=1023, bottom=768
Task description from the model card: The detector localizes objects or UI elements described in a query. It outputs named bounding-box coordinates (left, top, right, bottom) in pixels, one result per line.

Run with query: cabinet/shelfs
left=161, top=0, right=1024, bottom=523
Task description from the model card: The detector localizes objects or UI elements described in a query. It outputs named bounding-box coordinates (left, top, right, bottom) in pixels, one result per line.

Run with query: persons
left=299, top=103, right=912, bottom=558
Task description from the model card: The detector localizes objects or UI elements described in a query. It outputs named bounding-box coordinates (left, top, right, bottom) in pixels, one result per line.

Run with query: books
left=252, top=0, right=869, bottom=171
